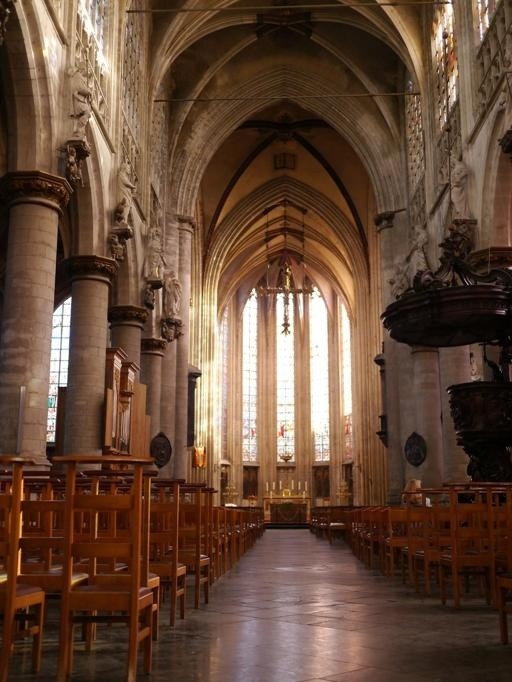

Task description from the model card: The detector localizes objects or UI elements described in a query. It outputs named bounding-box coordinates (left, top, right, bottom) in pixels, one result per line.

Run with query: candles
left=266, top=479, right=308, bottom=492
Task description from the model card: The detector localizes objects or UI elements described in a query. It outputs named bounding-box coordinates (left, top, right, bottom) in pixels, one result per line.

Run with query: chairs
left=309, top=482, right=511, bottom=647
left=1, top=455, right=266, bottom=681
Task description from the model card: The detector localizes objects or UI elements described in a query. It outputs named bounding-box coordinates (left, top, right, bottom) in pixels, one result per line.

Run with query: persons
left=144, top=214, right=164, bottom=279
left=164, top=268, right=182, bottom=320
left=401, top=479, right=431, bottom=507
left=114, top=162, right=137, bottom=226
left=69, top=65, right=92, bottom=137
left=446, top=153, right=473, bottom=219
left=409, top=223, right=430, bottom=278
